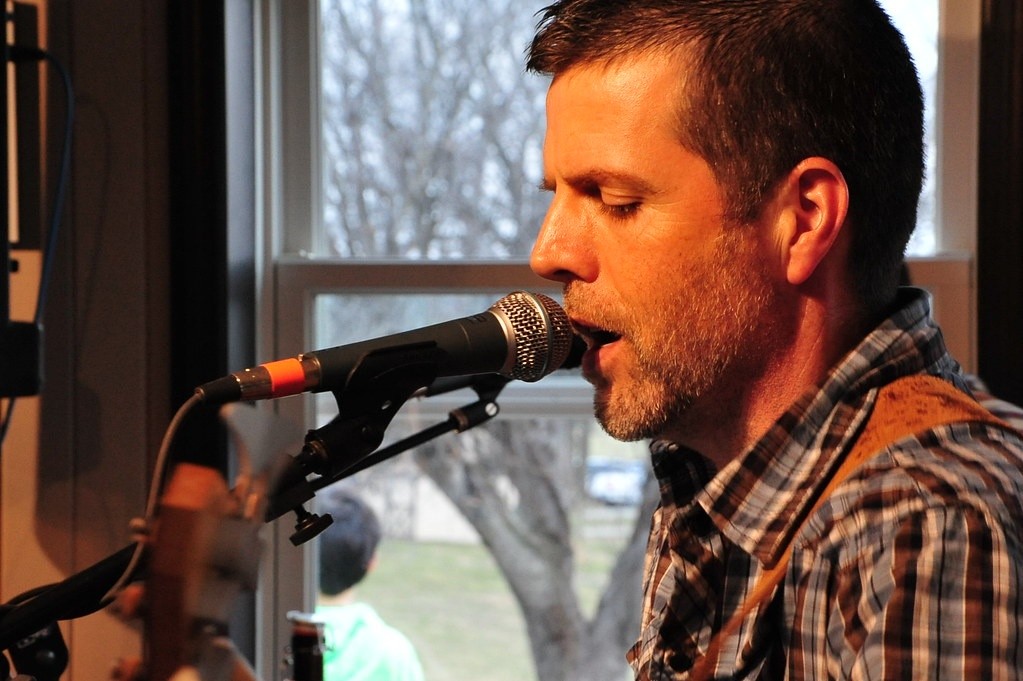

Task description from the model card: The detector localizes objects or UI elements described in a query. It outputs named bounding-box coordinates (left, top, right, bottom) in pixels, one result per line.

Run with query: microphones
left=195, top=289, right=577, bottom=409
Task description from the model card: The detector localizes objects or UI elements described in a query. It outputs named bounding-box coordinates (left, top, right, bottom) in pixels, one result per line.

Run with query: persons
left=523, top=1, right=1023, bottom=680
left=315, top=492, right=426, bottom=681
